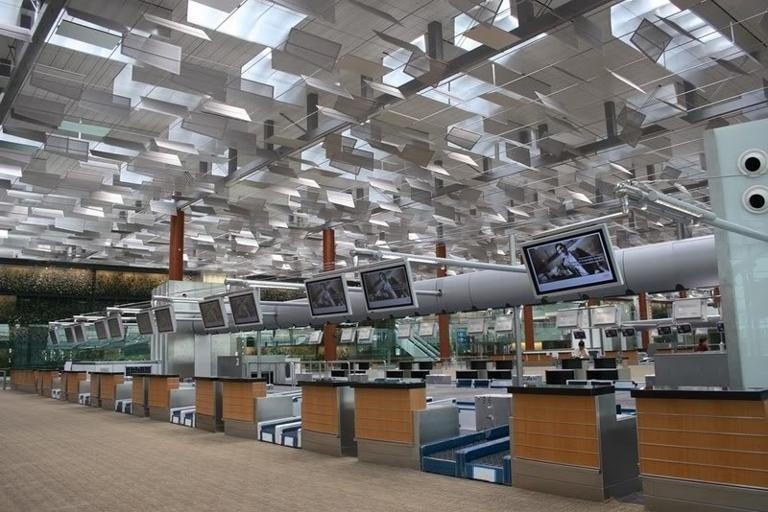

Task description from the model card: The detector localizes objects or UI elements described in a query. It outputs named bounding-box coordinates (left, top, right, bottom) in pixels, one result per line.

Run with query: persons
left=572, top=341, right=591, bottom=360
left=319, top=283, right=339, bottom=307
left=376, top=272, right=404, bottom=300
left=555, top=243, right=602, bottom=279
left=696, top=337, right=708, bottom=351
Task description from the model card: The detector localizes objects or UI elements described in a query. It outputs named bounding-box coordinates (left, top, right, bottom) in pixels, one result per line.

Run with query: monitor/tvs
left=517, top=222, right=624, bottom=300
left=359, top=261, right=419, bottom=314
left=558, top=299, right=725, bottom=339
left=198, top=296, right=229, bottom=331
left=303, top=272, right=352, bottom=321
left=257, top=314, right=512, bottom=347
left=229, top=291, right=263, bottom=327
left=49, top=306, right=176, bottom=346
